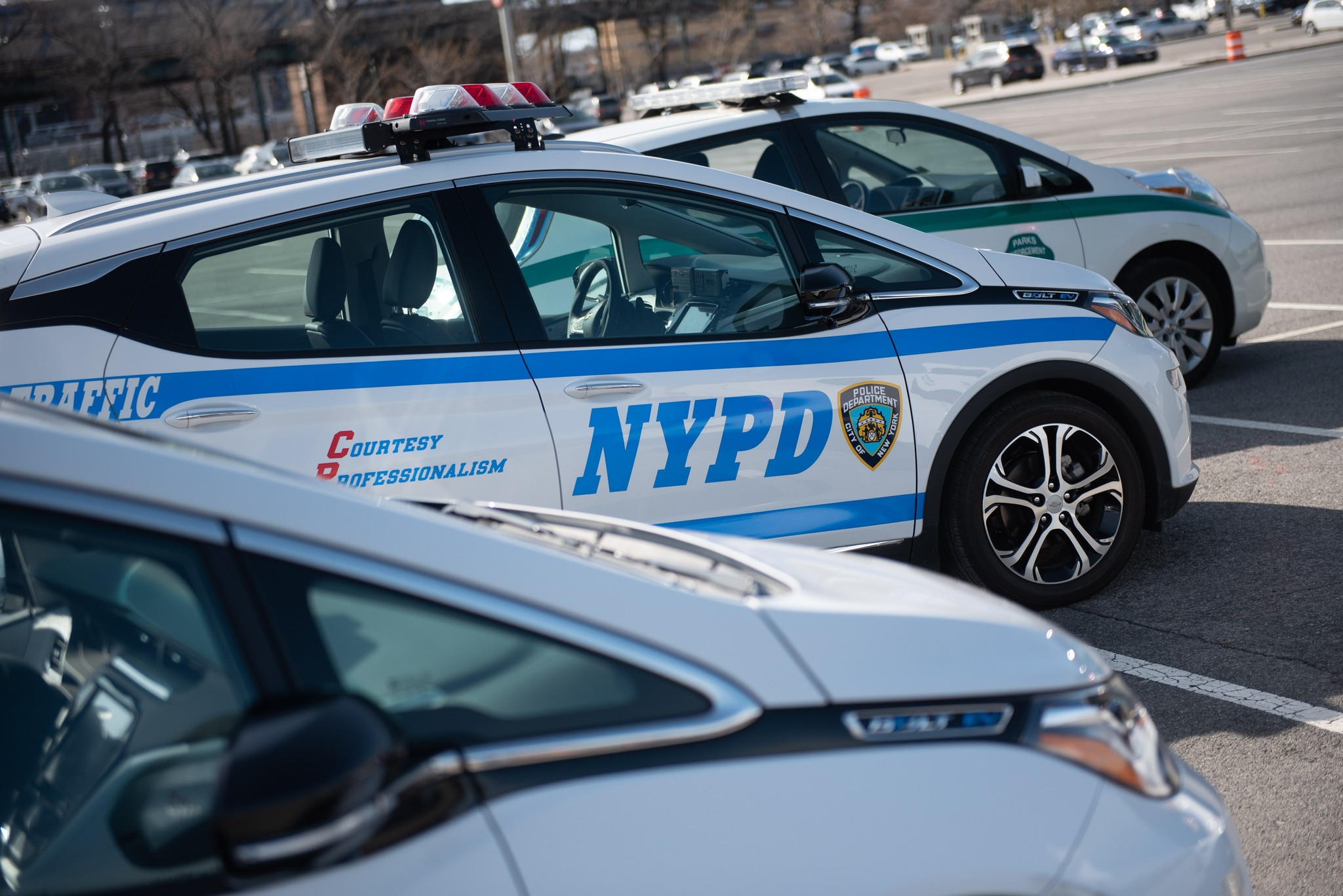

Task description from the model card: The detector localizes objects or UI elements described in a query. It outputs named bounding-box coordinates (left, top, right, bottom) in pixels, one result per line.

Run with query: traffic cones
left=852, top=87, right=872, bottom=132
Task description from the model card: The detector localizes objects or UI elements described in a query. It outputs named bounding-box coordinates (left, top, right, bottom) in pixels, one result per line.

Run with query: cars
left=400, top=71, right=1274, bottom=390
left=562, top=2, right=1343, bottom=124
left=0, top=80, right=1204, bottom=614
left=2, top=389, right=1245, bottom=896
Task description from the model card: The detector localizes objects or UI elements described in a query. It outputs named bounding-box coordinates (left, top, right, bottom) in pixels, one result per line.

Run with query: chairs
left=303, top=236, right=377, bottom=356
left=382, top=220, right=463, bottom=352
